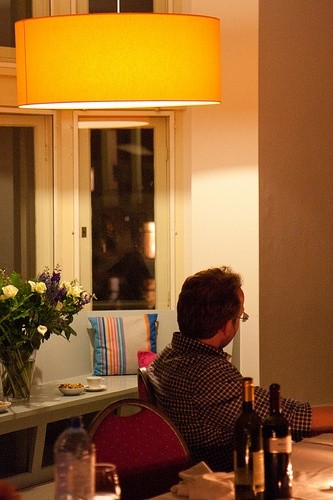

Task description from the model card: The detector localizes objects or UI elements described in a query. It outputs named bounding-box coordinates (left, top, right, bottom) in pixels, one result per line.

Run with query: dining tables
left=0, top=373, right=140, bottom=490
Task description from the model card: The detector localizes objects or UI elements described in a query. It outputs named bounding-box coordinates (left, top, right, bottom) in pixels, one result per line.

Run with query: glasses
left=232, top=312, right=249, bottom=322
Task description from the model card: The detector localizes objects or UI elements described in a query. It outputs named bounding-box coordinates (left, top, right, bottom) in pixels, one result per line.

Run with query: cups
left=85, top=377, right=105, bottom=388
left=91, top=463, right=122, bottom=500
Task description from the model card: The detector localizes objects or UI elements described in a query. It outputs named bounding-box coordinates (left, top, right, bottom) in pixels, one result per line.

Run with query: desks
left=148, top=432, right=333, bottom=500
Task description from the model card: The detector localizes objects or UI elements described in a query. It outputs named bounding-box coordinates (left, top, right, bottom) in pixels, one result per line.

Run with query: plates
left=85, top=384, right=106, bottom=391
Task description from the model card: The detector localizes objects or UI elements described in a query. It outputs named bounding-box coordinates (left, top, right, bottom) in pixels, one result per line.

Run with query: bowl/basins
left=0, top=401, right=11, bottom=413
left=58, top=387, right=84, bottom=395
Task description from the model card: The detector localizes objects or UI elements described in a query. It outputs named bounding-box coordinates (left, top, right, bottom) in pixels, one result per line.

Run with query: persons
left=146, top=267, right=333, bottom=472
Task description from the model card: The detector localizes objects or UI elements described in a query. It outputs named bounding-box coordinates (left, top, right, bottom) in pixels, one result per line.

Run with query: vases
left=0, top=350, right=37, bottom=402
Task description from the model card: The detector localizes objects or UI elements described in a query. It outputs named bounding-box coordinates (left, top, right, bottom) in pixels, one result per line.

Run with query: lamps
left=15, top=0, right=223, bottom=109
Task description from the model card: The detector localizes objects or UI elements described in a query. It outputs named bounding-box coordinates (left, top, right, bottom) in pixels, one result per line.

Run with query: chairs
left=87, top=398, right=190, bottom=500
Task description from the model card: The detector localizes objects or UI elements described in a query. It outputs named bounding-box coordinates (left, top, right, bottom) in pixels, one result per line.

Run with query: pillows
left=88, top=313, right=159, bottom=377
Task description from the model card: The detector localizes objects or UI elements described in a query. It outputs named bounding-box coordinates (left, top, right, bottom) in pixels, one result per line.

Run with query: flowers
left=0, top=264, right=97, bottom=350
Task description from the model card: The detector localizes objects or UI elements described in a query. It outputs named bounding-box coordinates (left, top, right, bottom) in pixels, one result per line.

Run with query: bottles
left=235, top=378, right=265, bottom=500
left=52, top=416, right=97, bottom=500
left=263, top=383, right=294, bottom=500
